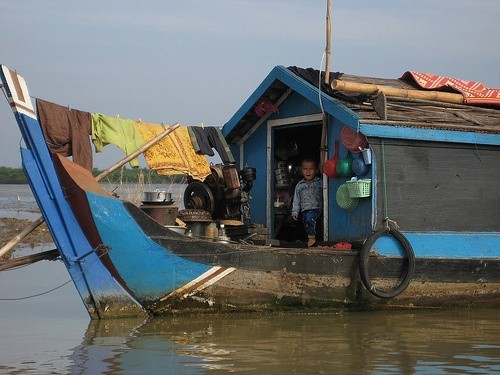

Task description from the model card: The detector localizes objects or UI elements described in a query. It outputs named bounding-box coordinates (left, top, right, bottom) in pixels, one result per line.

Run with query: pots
left=143, top=189, right=172, bottom=204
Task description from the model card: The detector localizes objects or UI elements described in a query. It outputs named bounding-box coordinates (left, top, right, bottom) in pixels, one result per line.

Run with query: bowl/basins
left=165, top=225, right=187, bottom=235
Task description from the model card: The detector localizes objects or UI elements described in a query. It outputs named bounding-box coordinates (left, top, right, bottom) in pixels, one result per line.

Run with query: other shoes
left=308, top=237, right=316, bottom=247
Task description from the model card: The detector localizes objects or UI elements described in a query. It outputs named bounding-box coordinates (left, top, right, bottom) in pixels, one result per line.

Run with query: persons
left=292, top=156, right=323, bottom=247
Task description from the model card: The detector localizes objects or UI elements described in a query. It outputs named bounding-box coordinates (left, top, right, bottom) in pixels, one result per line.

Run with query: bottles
left=218, top=224, right=226, bottom=239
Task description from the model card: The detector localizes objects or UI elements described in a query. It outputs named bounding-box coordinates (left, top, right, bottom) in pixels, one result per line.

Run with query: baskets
left=336, top=184, right=359, bottom=211
left=348, top=179, right=371, bottom=198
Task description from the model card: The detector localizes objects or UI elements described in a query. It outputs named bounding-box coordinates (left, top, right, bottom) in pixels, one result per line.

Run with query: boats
left=0, top=63, right=499, bottom=318
